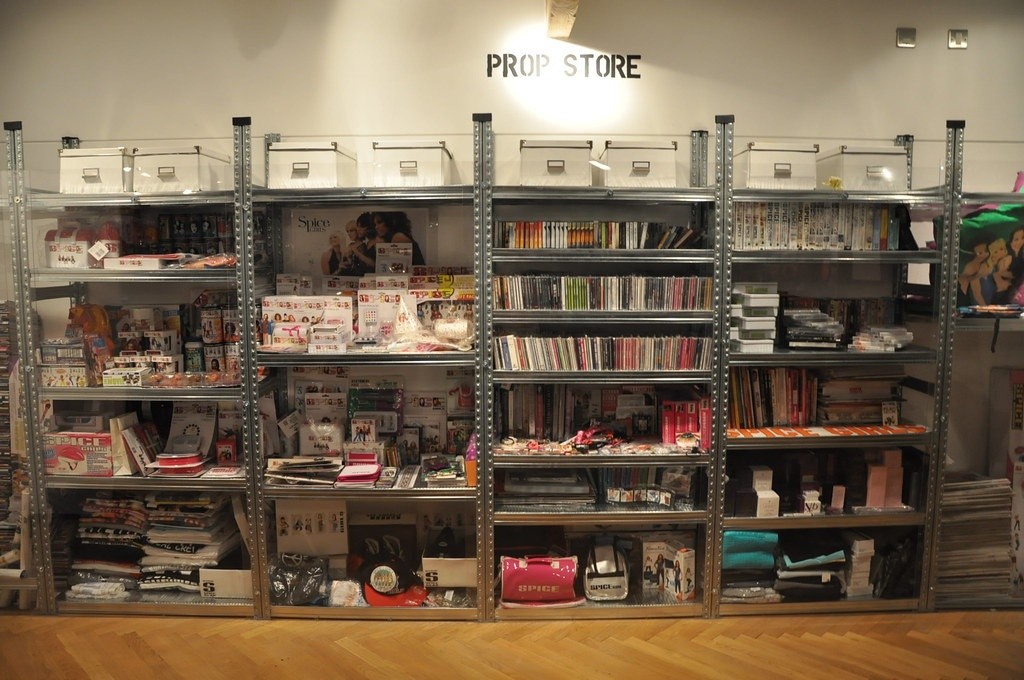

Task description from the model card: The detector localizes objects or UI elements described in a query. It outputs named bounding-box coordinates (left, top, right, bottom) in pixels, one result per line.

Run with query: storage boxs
left=631, top=529, right=698, bottom=603
left=732, top=141, right=820, bottom=190
left=261, top=243, right=475, bottom=355
left=730, top=280, right=781, bottom=356
left=597, top=140, right=679, bottom=189
left=41, top=429, right=113, bottom=478
left=35, top=328, right=187, bottom=389
left=101, top=254, right=169, bottom=271
left=57, top=146, right=138, bottom=196
left=131, top=144, right=231, bottom=195
left=814, top=144, right=909, bottom=192
left=724, top=447, right=906, bottom=519
left=419, top=525, right=478, bottom=589
left=370, top=138, right=454, bottom=189
left=266, top=139, right=358, bottom=190
left=841, top=528, right=876, bottom=598
left=273, top=496, right=350, bottom=580
left=516, top=139, right=594, bottom=188
left=276, top=379, right=449, bottom=465
left=200, top=563, right=254, bottom=600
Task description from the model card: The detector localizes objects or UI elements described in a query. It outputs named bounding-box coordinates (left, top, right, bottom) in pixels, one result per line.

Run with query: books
left=0, top=299, right=30, bottom=575
left=492, top=219, right=715, bottom=249
left=495, top=382, right=711, bottom=454
left=934, top=468, right=1017, bottom=604
left=774, top=293, right=914, bottom=345
left=490, top=271, right=712, bottom=313
left=727, top=202, right=911, bottom=251
left=492, top=332, right=711, bottom=371
left=729, top=366, right=905, bottom=430
left=496, top=466, right=705, bottom=506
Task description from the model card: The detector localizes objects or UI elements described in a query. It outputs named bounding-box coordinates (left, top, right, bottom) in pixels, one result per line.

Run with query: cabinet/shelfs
left=0, top=111, right=1024, bottom=622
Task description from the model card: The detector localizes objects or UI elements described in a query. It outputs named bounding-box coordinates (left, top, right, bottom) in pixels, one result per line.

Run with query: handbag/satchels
left=500, top=554, right=578, bottom=602
left=583, top=543, right=628, bottom=601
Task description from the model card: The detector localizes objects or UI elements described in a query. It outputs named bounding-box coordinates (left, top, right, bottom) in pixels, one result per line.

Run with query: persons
left=643, top=554, right=694, bottom=594
left=320, top=210, right=427, bottom=277
